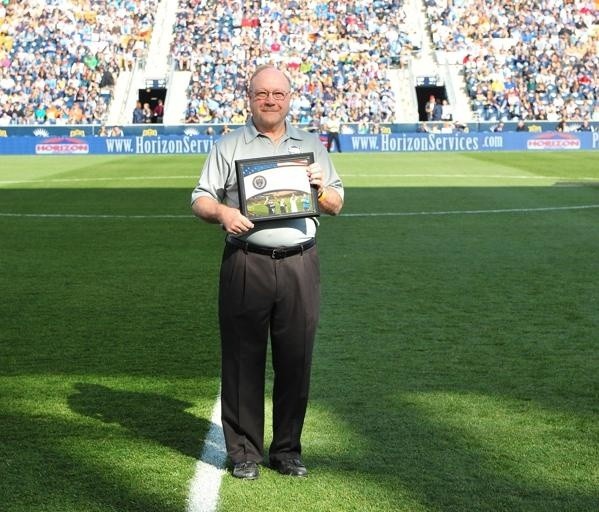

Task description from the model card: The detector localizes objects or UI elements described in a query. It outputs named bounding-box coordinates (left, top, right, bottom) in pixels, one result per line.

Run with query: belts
left=225, top=232, right=316, bottom=260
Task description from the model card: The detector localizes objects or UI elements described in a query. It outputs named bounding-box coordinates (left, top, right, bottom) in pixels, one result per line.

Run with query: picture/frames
left=234, top=152, right=320, bottom=223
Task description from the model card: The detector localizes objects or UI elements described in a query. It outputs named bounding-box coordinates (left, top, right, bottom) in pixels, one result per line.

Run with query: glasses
left=247, top=86, right=292, bottom=101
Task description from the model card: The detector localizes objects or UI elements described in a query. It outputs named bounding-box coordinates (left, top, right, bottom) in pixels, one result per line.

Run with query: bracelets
left=318, top=187, right=327, bottom=202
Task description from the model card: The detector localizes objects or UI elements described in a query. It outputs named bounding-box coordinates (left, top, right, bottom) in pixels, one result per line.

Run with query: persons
left=289, top=193, right=299, bottom=212
left=276, top=197, right=288, bottom=212
left=322, top=111, right=339, bottom=152
left=301, top=192, right=312, bottom=212
left=189, top=64, right=347, bottom=481
left=1, top=1, right=599, bottom=134
left=263, top=196, right=277, bottom=215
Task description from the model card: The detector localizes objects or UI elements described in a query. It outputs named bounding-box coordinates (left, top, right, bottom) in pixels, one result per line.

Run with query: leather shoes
left=268, top=456, right=308, bottom=478
left=233, top=460, right=259, bottom=480
